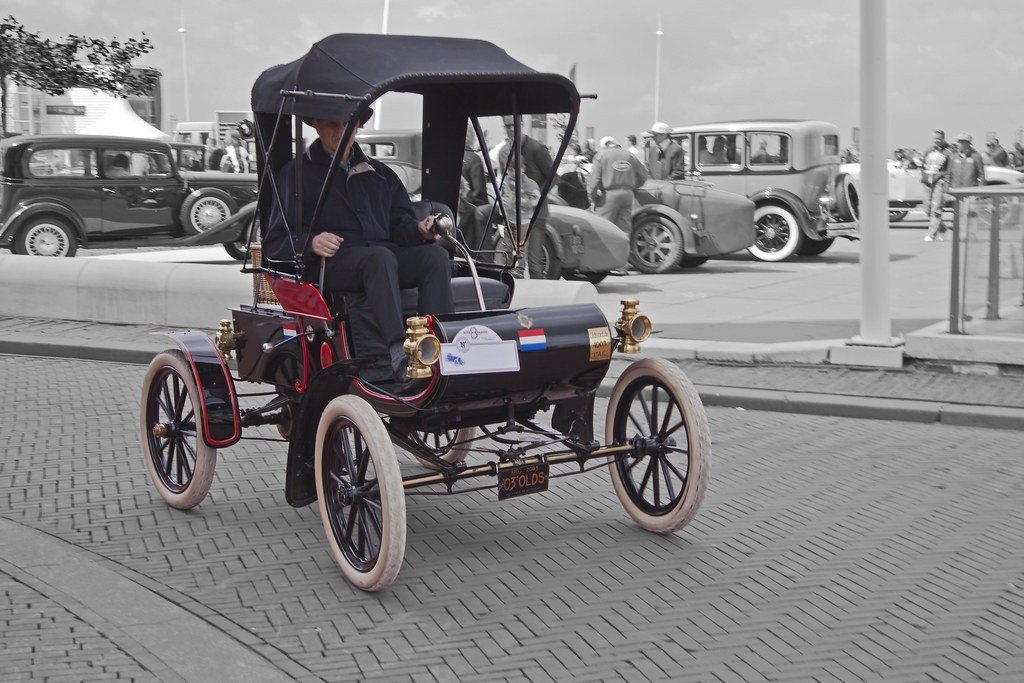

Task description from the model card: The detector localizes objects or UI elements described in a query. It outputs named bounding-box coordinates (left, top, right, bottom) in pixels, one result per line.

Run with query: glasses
left=504, top=123, right=515, bottom=128
left=643, top=137, right=648, bottom=139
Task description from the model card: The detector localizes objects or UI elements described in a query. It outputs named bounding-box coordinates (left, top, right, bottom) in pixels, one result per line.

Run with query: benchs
left=403, top=198, right=514, bottom=318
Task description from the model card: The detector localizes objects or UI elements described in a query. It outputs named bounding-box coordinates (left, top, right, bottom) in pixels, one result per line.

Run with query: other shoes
left=939, top=228, right=948, bottom=240
left=608, top=268, right=629, bottom=276
left=391, top=357, right=437, bottom=396
left=924, top=236, right=934, bottom=243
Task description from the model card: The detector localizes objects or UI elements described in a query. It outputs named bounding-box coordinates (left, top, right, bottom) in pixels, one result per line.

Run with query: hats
left=647, top=122, right=672, bottom=134
left=641, top=132, right=653, bottom=137
left=957, top=132, right=973, bottom=144
left=301, top=106, right=374, bottom=128
left=933, top=130, right=944, bottom=134
left=600, top=136, right=616, bottom=147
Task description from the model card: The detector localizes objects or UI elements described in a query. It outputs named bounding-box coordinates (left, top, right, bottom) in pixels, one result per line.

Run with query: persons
left=263, top=108, right=458, bottom=395
left=109, top=130, right=252, bottom=177
left=843, top=131, right=1024, bottom=244
left=462, top=113, right=769, bottom=276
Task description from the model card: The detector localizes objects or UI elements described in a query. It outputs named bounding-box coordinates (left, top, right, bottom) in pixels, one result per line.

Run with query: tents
left=76, top=100, right=167, bottom=140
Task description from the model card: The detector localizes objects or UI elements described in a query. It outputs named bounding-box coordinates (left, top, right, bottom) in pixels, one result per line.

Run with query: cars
left=1, top=133, right=261, bottom=260
left=140, top=31, right=713, bottom=595
left=638, top=117, right=860, bottom=262
left=840, top=156, right=1024, bottom=230
left=169, top=110, right=756, bottom=284
